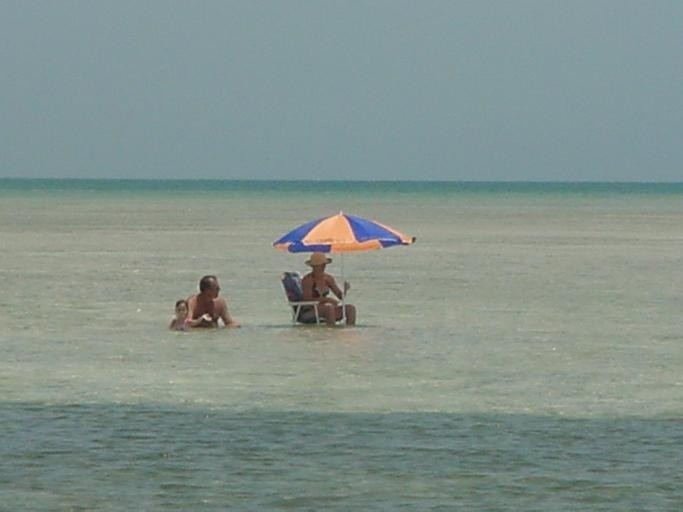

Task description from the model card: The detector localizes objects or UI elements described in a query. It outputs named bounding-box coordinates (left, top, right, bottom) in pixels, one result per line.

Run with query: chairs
left=279, top=271, right=347, bottom=327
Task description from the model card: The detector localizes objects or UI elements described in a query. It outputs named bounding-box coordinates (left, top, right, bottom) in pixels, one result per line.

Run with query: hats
left=305, top=252, right=331, bottom=267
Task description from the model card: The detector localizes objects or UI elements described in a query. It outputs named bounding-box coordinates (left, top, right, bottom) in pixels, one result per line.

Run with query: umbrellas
left=274, top=209, right=416, bottom=323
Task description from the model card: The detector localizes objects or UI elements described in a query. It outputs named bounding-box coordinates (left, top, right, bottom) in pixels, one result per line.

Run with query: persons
left=295, top=251, right=356, bottom=327
left=186, top=273, right=240, bottom=327
left=169, top=299, right=190, bottom=329
